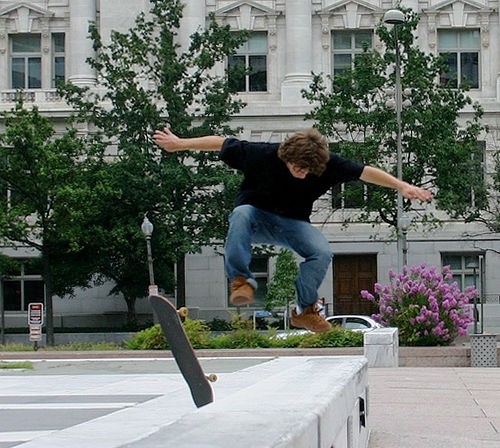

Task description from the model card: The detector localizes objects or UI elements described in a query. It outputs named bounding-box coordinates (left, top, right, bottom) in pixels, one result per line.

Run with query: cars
left=275, top=314, right=387, bottom=342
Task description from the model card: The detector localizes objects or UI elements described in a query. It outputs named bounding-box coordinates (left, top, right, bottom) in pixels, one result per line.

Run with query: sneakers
left=230, top=278, right=255, bottom=306
left=291, top=306, right=332, bottom=333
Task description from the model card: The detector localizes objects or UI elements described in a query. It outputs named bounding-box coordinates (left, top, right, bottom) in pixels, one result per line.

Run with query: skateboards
left=149, top=295, right=217, bottom=408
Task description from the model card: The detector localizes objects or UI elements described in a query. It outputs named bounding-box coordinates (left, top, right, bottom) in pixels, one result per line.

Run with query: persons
left=153, top=127, right=432, bottom=333
